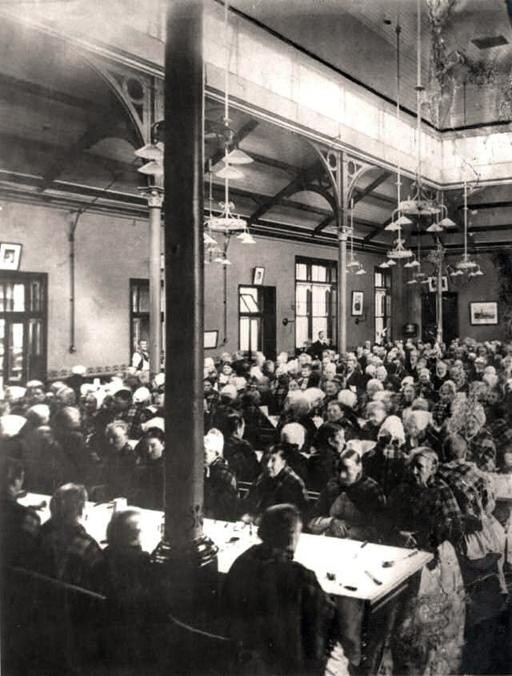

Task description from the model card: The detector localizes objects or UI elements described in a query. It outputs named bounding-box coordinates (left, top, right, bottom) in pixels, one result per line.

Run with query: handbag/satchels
left=458, top=531, right=509, bottom=628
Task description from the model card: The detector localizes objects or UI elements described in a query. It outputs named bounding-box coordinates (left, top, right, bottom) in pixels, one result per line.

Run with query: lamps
left=133, top=0, right=489, bottom=286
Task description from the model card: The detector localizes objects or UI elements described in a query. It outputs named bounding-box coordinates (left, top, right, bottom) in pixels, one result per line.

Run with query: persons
left=2, top=331, right=510, bottom=675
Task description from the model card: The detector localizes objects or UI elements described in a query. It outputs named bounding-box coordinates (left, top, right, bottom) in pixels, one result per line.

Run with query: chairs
left=4, top=563, right=256, bottom=674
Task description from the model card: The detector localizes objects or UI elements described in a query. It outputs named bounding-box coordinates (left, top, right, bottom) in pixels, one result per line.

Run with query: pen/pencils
left=365, top=569, right=382, bottom=586
left=403, top=551, right=418, bottom=560
left=360, top=540, right=370, bottom=549
left=249, top=522, right=253, bottom=536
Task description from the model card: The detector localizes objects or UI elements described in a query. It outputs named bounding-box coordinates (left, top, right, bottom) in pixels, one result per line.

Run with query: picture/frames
left=350, top=289, right=364, bottom=317
left=251, top=267, right=266, bottom=285
left=467, top=299, right=500, bottom=326
left=0, top=239, right=24, bottom=272
left=203, top=329, right=218, bottom=350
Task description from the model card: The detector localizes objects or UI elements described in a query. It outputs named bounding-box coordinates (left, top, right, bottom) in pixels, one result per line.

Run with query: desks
left=19, top=491, right=434, bottom=676
